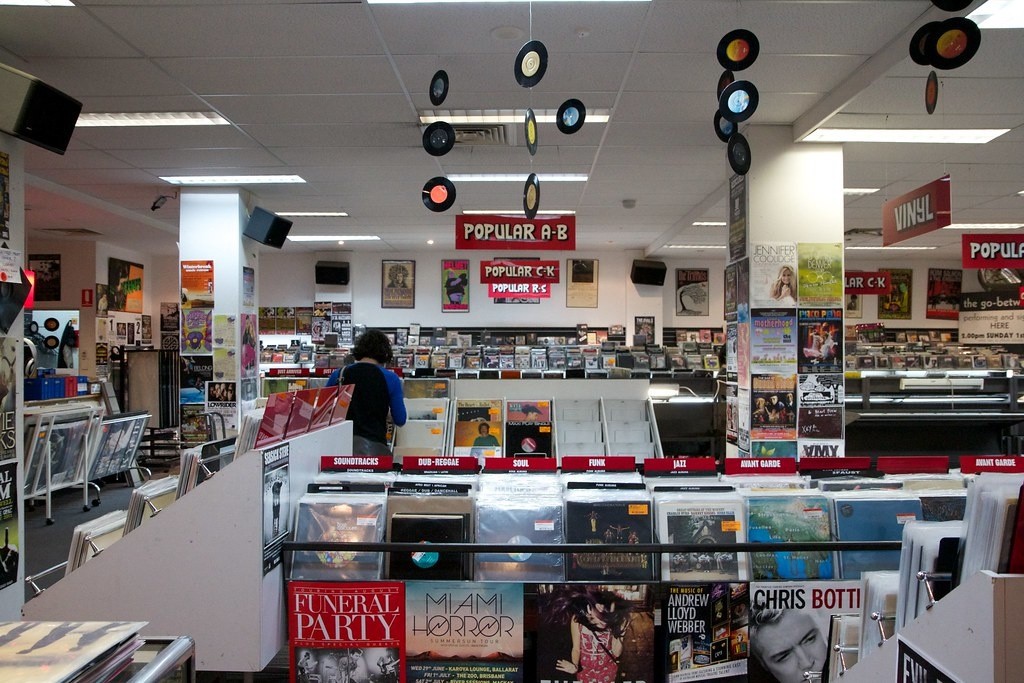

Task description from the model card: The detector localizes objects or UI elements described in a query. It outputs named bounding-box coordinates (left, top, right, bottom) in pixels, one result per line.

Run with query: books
left=64, top=397, right=269, bottom=578
left=289, top=379, right=1024, bottom=683
left=0, top=622, right=150, bottom=682
left=261, top=324, right=727, bottom=372
left=847, top=330, right=1022, bottom=374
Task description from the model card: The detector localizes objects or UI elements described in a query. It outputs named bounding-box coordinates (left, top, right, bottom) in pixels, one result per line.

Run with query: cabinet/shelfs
left=0, top=325, right=1024, bottom=683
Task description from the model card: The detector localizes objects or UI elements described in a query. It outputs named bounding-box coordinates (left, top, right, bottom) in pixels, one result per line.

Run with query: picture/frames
left=493, top=257, right=540, bottom=304
left=381, top=259, right=416, bottom=309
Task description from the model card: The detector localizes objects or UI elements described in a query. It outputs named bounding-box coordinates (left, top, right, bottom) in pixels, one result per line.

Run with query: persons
left=297, top=652, right=313, bottom=676
left=749, top=599, right=827, bottom=683
left=555, top=591, right=630, bottom=683
left=522, top=405, right=542, bottom=421
left=336, top=648, right=362, bottom=683
left=326, top=354, right=355, bottom=385
left=325, top=328, right=407, bottom=458
left=754, top=393, right=794, bottom=425
left=378, top=649, right=396, bottom=682
left=242, top=334, right=255, bottom=367
left=470, top=423, right=499, bottom=458
left=771, top=266, right=797, bottom=303
left=892, top=282, right=908, bottom=312
left=804, top=330, right=834, bottom=364
left=241, top=316, right=255, bottom=344
